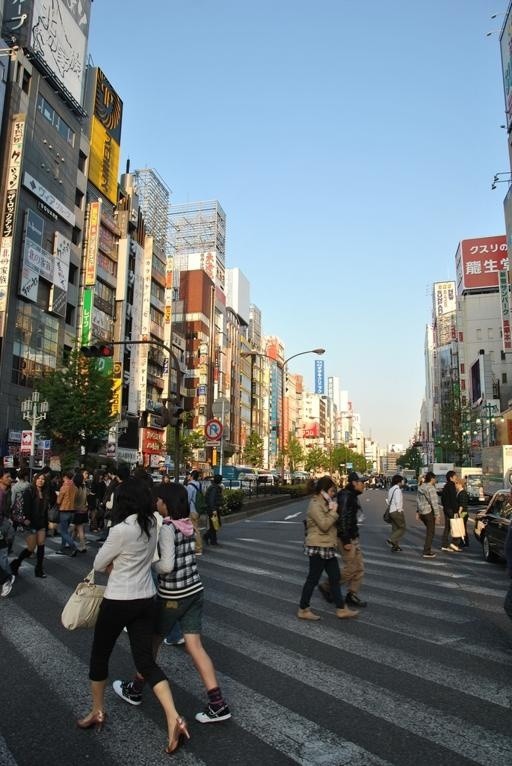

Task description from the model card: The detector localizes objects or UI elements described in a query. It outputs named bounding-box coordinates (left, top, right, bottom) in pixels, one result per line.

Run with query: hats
left=348, top=471, right=370, bottom=482
left=187, top=470, right=199, bottom=478
left=392, top=475, right=403, bottom=485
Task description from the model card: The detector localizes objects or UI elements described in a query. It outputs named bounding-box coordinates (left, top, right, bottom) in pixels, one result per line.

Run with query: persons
left=77, top=480, right=191, bottom=754
left=297, top=477, right=360, bottom=620
left=318, top=471, right=369, bottom=607
left=112, top=483, right=233, bottom=724
left=1, top=461, right=222, bottom=599
left=441, top=471, right=469, bottom=552
left=385, top=475, right=406, bottom=552
left=504, top=522, right=512, bottom=652
left=415, top=471, right=438, bottom=558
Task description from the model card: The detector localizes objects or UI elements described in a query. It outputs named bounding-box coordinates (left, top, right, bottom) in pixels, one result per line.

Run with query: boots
left=335, top=605, right=359, bottom=618
left=298, top=607, right=320, bottom=620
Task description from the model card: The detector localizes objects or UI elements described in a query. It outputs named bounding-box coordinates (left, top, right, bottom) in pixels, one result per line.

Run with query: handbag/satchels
left=384, top=506, right=392, bottom=524
left=450, top=517, right=466, bottom=538
left=61, top=582, right=107, bottom=631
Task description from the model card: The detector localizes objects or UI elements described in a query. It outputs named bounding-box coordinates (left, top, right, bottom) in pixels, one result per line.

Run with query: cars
left=474, top=488, right=512, bottom=562
left=152, top=466, right=282, bottom=492
left=349, top=472, right=400, bottom=489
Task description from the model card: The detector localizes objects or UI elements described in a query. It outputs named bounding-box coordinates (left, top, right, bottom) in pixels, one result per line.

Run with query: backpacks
left=186, top=481, right=208, bottom=515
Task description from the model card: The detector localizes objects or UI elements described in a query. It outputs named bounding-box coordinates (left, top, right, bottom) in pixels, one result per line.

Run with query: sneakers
left=423, top=552, right=436, bottom=558
left=318, top=584, right=366, bottom=606
left=163, top=637, right=184, bottom=645
left=195, top=702, right=232, bottom=724
left=385, top=538, right=403, bottom=552
left=0, top=574, right=16, bottom=597
left=34, top=568, right=46, bottom=577
left=112, top=680, right=142, bottom=706
left=10, top=560, right=18, bottom=575
left=202, top=534, right=222, bottom=548
left=55, top=543, right=86, bottom=557
left=441, top=543, right=464, bottom=552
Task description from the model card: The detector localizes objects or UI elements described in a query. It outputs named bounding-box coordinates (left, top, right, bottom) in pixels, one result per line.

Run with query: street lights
left=307, top=414, right=356, bottom=474
left=239, top=348, right=326, bottom=481
left=429, top=399, right=506, bottom=461
left=332, top=435, right=360, bottom=474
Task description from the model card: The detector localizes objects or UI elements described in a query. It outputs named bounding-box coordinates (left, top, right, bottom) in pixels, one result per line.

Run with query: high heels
left=76, top=711, right=106, bottom=734
left=164, top=715, right=191, bottom=754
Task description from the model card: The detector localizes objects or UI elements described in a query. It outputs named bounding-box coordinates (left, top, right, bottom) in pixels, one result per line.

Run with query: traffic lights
left=153, top=406, right=168, bottom=426
left=80, top=344, right=114, bottom=357
left=170, top=406, right=184, bottom=426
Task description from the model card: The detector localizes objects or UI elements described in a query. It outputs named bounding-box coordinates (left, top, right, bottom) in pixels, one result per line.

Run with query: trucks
left=401, top=446, right=512, bottom=498
